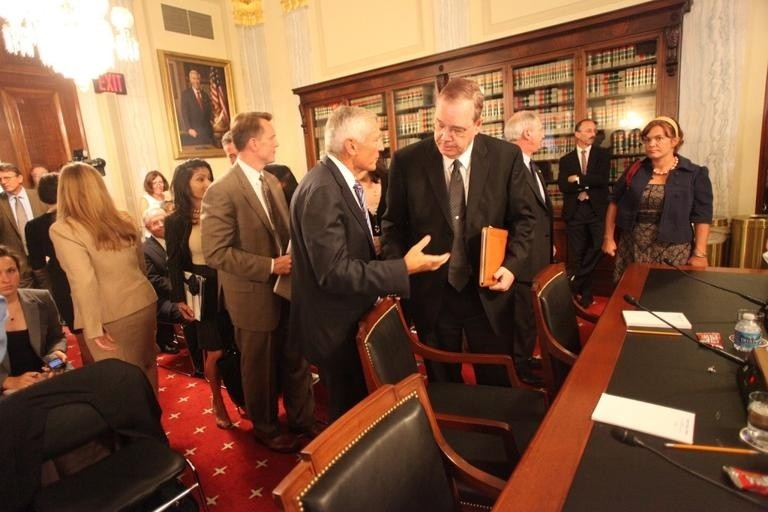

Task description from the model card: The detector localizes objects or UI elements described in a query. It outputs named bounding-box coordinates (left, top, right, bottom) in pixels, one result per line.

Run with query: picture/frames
left=155, top=45, right=239, bottom=161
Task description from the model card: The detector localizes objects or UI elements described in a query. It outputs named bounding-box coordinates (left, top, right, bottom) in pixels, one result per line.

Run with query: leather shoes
left=158, top=340, right=179, bottom=353
left=517, top=355, right=545, bottom=388
left=579, top=297, right=593, bottom=308
left=293, top=422, right=322, bottom=437
left=254, top=430, right=299, bottom=453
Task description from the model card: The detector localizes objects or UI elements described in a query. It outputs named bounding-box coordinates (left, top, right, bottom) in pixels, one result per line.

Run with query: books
left=315, top=46, right=658, bottom=289
left=590, top=394, right=696, bottom=446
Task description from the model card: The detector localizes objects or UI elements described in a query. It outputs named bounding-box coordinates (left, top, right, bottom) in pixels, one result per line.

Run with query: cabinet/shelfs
left=288, top=0, right=698, bottom=267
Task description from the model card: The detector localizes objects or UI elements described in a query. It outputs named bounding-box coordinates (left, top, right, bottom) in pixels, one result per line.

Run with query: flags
left=208, top=67, right=231, bottom=127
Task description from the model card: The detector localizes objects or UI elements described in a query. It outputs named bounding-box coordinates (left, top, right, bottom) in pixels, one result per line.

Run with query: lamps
left=1, top=0, right=139, bottom=87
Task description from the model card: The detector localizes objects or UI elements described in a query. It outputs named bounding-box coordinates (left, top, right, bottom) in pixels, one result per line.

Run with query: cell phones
left=48, top=356, right=66, bottom=371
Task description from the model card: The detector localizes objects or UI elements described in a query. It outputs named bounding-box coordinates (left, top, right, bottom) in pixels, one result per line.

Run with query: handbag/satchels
left=216, top=348, right=244, bottom=408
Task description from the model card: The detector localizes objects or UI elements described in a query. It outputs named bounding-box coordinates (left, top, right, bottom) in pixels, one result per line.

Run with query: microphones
left=622, top=293, right=746, bottom=364
left=611, top=426, right=768, bottom=512
left=663, top=259, right=768, bottom=306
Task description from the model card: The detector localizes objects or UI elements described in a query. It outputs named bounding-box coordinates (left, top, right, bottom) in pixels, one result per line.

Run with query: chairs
left=266, top=370, right=511, bottom=512
left=2, top=357, right=208, bottom=511
left=523, top=259, right=599, bottom=397
left=354, top=294, right=545, bottom=471
left=154, top=318, right=197, bottom=377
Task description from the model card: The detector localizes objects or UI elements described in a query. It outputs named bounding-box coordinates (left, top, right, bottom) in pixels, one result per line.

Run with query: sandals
left=212, top=397, right=231, bottom=428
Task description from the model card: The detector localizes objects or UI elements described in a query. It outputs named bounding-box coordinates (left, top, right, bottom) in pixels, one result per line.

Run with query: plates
left=728, top=334, right=768, bottom=348
left=736, top=426, right=767, bottom=452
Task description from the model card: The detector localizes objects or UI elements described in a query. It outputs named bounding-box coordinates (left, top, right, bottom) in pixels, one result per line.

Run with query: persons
left=288, top=77, right=715, bottom=424
left=180, top=72, right=215, bottom=146
left=1, top=112, right=330, bottom=454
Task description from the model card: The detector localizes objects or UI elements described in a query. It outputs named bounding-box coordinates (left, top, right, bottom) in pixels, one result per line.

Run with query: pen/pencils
left=626, top=330, right=683, bottom=336
left=664, top=443, right=759, bottom=455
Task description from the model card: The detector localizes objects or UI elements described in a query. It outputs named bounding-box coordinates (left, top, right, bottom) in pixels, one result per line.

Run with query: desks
left=492, top=261, right=767, bottom=509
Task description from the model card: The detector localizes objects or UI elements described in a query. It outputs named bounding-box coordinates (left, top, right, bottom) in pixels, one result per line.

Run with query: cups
left=736, top=308, right=764, bottom=332
left=745, top=390, right=768, bottom=445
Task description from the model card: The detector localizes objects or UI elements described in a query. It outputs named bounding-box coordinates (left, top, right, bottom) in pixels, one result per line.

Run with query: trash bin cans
left=691, top=214, right=768, bottom=269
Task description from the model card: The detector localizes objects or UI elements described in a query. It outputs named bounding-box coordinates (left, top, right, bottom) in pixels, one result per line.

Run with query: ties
left=196, top=91, right=206, bottom=112
left=260, top=176, right=289, bottom=256
left=580, top=151, right=587, bottom=175
left=354, top=184, right=375, bottom=243
left=14, top=196, right=30, bottom=257
left=447, top=160, right=472, bottom=293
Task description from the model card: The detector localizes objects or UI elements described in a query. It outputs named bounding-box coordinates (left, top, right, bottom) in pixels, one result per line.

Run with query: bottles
left=732, top=315, right=761, bottom=351
